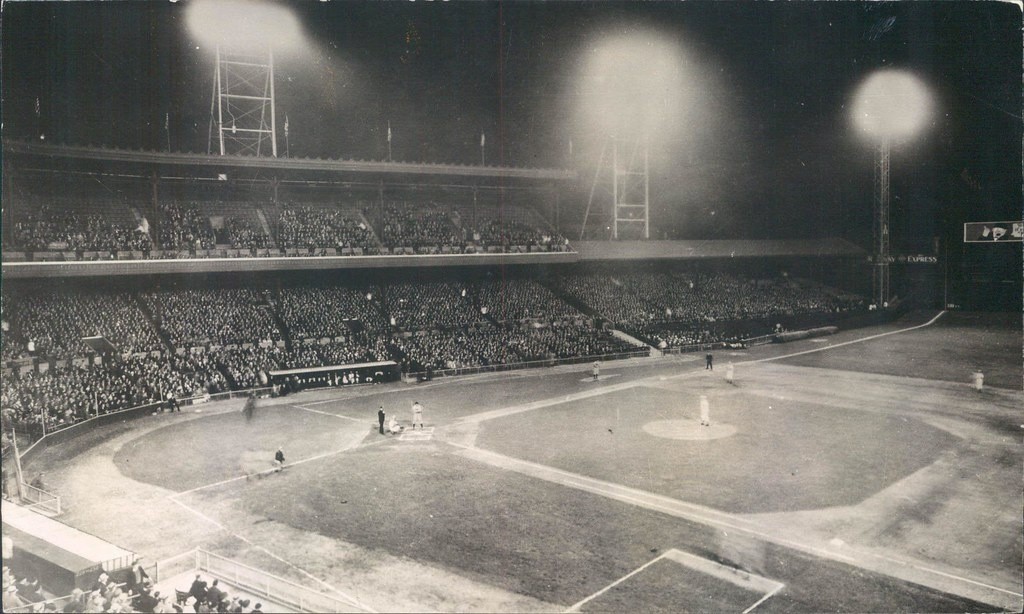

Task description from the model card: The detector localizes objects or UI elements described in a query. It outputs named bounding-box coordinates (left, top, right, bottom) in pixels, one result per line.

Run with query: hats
left=185, top=596, right=197, bottom=606
left=2, top=566, right=10, bottom=572
left=98, top=573, right=109, bottom=582
left=8, top=586, right=19, bottom=592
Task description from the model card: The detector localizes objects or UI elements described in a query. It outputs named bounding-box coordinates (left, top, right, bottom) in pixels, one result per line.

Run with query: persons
left=725, top=361, right=734, bottom=383
left=0, top=528, right=264, bottom=614
left=705, top=350, right=713, bottom=371
left=377, top=405, right=385, bottom=434
left=274, top=449, right=285, bottom=471
left=592, top=358, right=600, bottom=379
left=412, top=401, right=424, bottom=430
left=0, top=182, right=902, bottom=492
left=699, top=395, right=710, bottom=426
left=974, top=369, right=984, bottom=393
left=168, top=395, right=180, bottom=412
left=388, top=415, right=401, bottom=434
